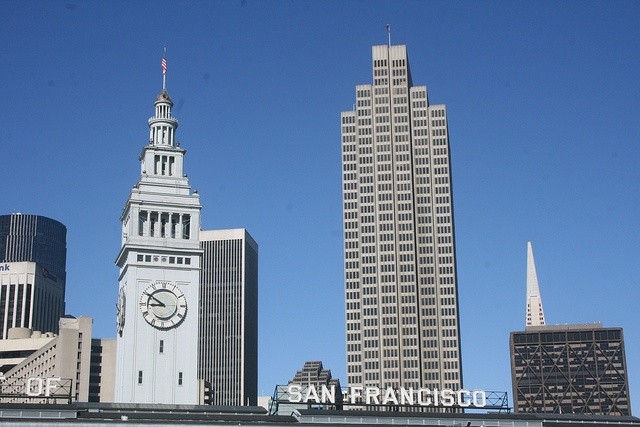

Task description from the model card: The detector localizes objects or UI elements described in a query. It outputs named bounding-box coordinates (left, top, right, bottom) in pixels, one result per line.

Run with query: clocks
left=139, top=281, right=187, bottom=329
